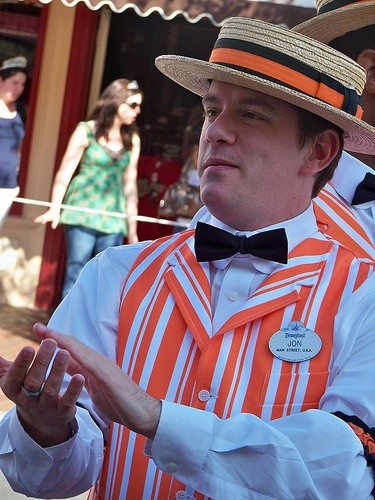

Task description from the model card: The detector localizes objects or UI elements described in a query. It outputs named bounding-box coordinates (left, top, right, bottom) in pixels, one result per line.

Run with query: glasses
left=125, top=102, right=141, bottom=108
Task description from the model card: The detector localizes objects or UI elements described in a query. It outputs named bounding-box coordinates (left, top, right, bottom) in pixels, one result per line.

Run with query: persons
left=0, top=17, right=375, bottom=500
left=34, top=79, right=142, bottom=301
left=290, top=0, right=375, bottom=262
left=0, top=51, right=27, bottom=224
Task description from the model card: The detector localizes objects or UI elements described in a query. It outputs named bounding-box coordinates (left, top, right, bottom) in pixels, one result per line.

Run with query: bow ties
left=351, top=172, right=375, bottom=206
left=195, top=221, right=288, bottom=264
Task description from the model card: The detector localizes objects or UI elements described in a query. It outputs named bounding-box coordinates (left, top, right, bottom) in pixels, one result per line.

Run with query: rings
left=21, top=383, right=42, bottom=398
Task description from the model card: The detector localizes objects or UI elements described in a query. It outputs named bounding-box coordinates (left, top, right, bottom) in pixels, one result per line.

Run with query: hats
left=290, top=0, right=375, bottom=44
left=155, top=17, right=375, bottom=155
